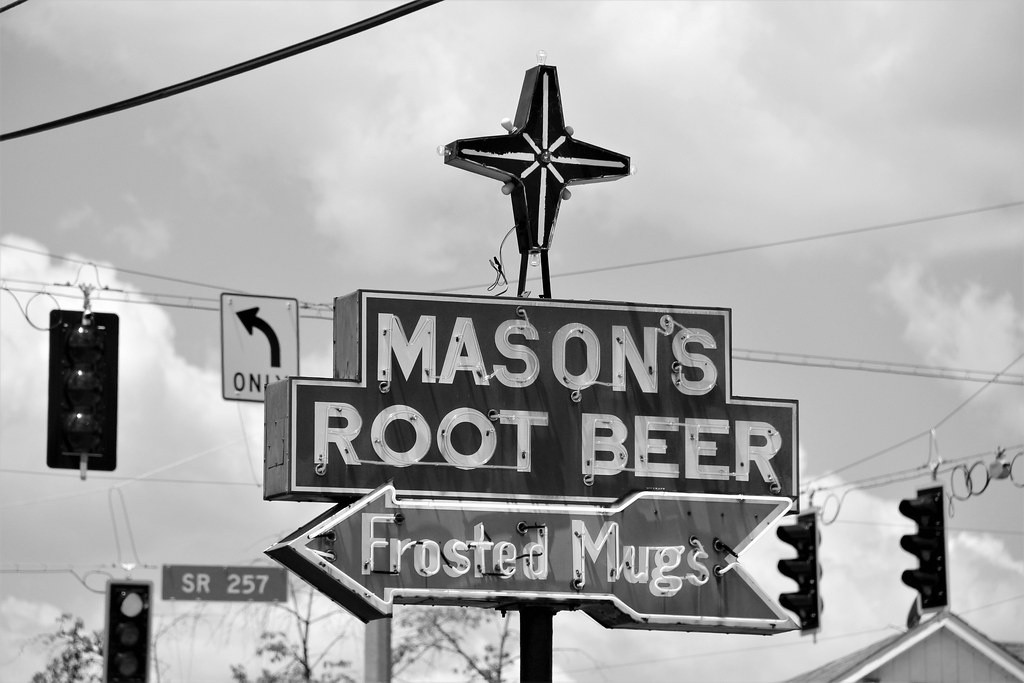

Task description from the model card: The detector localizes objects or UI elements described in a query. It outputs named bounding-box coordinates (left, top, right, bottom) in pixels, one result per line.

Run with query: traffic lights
left=776, top=512, right=819, bottom=630
left=104, top=578, right=153, bottom=683
left=899, top=487, right=948, bottom=610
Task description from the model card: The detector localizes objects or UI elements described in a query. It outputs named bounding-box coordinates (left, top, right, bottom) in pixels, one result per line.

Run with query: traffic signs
left=262, top=476, right=794, bottom=637
